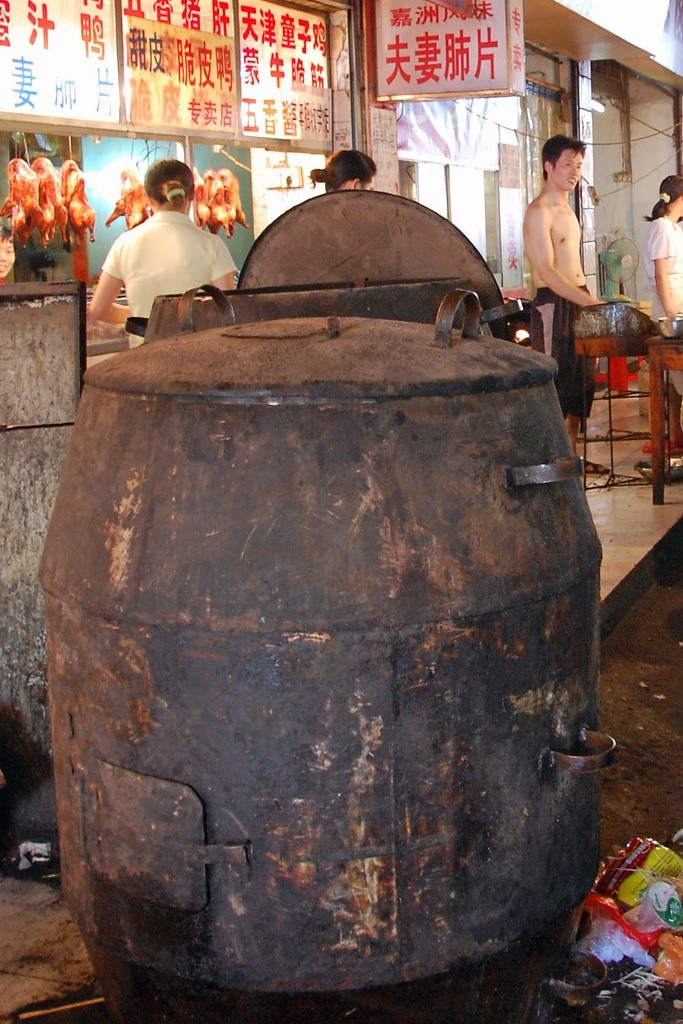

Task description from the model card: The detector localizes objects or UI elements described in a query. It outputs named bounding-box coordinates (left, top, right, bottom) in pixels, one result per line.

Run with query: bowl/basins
left=633, top=457, right=683, bottom=481
left=658, top=316, right=683, bottom=337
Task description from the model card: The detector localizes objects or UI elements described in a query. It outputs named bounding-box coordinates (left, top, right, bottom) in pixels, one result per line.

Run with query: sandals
left=585, top=460, right=611, bottom=475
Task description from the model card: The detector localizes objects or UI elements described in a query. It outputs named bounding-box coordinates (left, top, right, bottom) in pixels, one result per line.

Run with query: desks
left=620, top=301, right=652, bottom=319
left=644, top=334, right=683, bottom=504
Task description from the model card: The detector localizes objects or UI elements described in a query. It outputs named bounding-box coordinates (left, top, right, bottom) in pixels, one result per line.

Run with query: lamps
left=591, top=94, right=606, bottom=113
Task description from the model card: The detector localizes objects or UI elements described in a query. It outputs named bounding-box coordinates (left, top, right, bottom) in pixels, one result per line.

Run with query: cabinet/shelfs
left=575, top=343, right=671, bottom=488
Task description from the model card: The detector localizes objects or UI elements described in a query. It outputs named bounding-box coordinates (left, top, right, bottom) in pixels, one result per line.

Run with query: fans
left=598, top=237, right=641, bottom=302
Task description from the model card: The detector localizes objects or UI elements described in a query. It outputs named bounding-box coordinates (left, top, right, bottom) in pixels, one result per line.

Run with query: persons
left=522, top=134, right=609, bottom=473
left=0, top=224, right=16, bottom=286
left=90, top=157, right=237, bottom=350
left=642, top=175, right=683, bottom=455
left=311, top=149, right=376, bottom=193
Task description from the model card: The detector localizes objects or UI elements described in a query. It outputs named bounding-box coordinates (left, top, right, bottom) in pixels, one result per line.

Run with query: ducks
left=106, top=167, right=251, bottom=240
left=0, top=157, right=96, bottom=250
